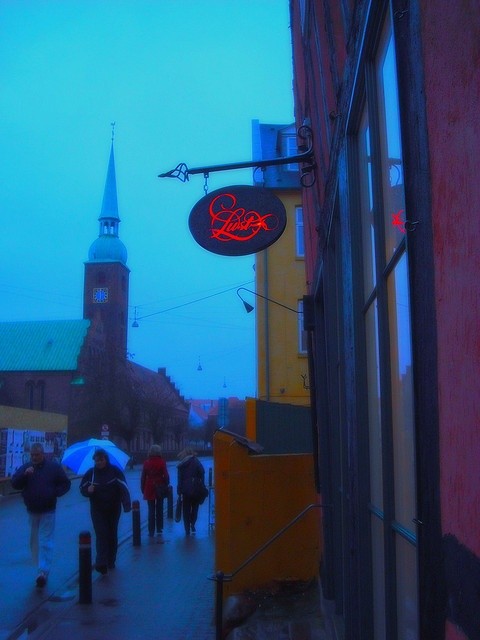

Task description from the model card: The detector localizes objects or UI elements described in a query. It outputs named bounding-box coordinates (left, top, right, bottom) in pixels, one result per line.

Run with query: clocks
left=93, top=289, right=109, bottom=304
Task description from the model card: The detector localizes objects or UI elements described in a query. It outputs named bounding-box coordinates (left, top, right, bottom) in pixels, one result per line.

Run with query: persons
left=140, top=442, right=169, bottom=544
left=176, top=447, right=208, bottom=535
left=12, top=443, right=72, bottom=586
left=80, top=450, right=132, bottom=576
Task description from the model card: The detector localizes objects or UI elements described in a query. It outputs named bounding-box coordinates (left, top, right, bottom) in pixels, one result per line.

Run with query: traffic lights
left=100, top=423, right=113, bottom=440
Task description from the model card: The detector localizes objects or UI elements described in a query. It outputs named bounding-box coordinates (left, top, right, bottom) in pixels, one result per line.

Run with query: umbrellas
left=61, top=438, right=130, bottom=478
